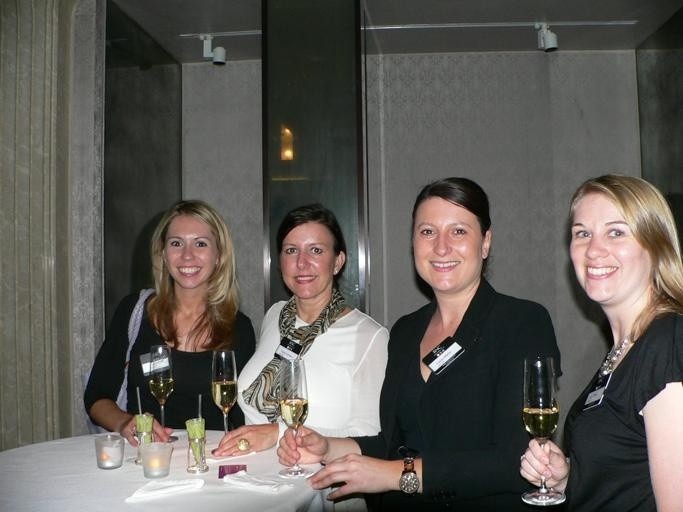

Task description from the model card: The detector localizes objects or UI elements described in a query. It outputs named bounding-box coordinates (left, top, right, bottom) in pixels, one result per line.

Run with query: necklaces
left=599, top=338, right=628, bottom=377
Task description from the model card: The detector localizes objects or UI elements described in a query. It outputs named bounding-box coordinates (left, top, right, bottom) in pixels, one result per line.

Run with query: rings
left=236, top=438, right=249, bottom=452
left=520, top=455, right=526, bottom=460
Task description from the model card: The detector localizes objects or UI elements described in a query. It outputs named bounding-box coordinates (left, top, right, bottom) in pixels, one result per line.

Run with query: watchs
left=399, top=456, right=420, bottom=494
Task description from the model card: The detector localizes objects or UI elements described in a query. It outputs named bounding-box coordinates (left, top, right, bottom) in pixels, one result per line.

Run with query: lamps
left=538, top=25, right=559, bottom=51
left=202, top=33, right=226, bottom=67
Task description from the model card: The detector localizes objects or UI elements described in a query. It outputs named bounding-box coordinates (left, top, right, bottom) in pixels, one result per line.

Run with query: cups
left=94, top=434, right=125, bottom=470
left=141, top=440, right=175, bottom=480
left=134, top=414, right=155, bottom=466
left=183, top=416, right=209, bottom=475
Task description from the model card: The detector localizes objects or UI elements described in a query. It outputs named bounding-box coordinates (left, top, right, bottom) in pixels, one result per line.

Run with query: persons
left=277, top=178, right=563, bottom=512
left=212, top=203, right=389, bottom=457
left=83, top=200, right=256, bottom=446
left=518, top=170, right=683, bottom=512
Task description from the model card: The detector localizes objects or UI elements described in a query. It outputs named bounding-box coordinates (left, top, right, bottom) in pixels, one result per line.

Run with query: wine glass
left=275, top=358, right=316, bottom=481
left=518, top=355, right=568, bottom=508
left=209, top=347, right=240, bottom=456
left=148, top=343, right=181, bottom=444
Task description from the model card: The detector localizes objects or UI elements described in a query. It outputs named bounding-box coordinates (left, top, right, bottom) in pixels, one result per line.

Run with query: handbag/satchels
left=86, top=288, right=156, bottom=433
